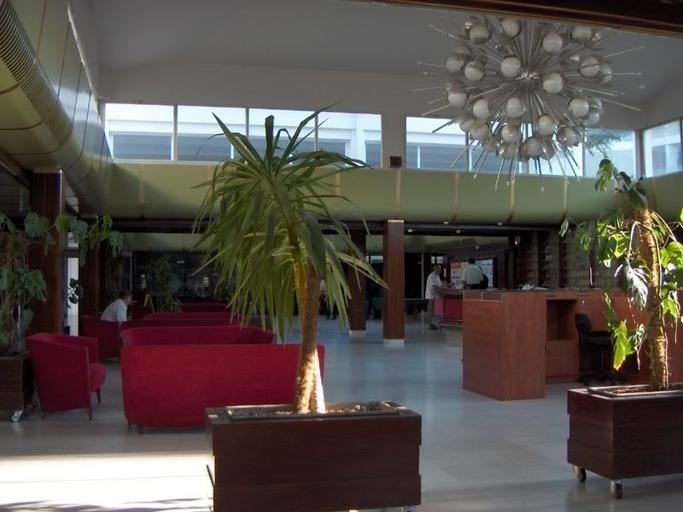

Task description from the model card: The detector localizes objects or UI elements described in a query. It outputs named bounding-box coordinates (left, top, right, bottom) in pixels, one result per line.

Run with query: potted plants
left=0, top=213, right=77, bottom=423
left=64, top=213, right=124, bottom=335
left=557, top=159, right=683, bottom=500
left=187, top=102, right=423, bottom=511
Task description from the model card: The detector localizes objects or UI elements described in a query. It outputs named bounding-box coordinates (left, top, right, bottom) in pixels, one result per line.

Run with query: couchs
left=117, top=303, right=324, bottom=435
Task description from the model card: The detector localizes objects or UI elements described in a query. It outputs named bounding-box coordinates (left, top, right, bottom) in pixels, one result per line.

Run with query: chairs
left=80, top=315, right=123, bottom=360
left=574, top=312, right=631, bottom=387
left=28, top=331, right=107, bottom=421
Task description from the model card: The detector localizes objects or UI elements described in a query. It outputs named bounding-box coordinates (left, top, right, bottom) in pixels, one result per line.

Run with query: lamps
left=410, top=12, right=646, bottom=192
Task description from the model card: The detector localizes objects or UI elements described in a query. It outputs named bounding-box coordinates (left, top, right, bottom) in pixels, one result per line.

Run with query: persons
left=100, top=288, right=129, bottom=322
left=470, top=267, right=488, bottom=289
left=140, top=272, right=155, bottom=313
left=425, top=265, right=442, bottom=329
left=461, top=257, right=484, bottom=289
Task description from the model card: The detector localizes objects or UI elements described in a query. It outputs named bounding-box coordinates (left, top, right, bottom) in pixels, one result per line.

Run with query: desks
left=460, top=288, right=683, bottom=400
